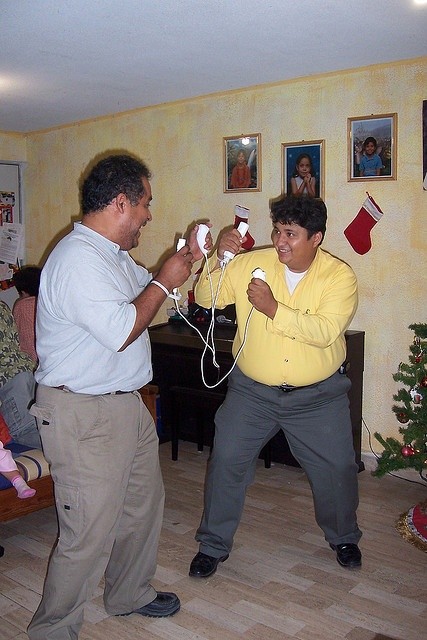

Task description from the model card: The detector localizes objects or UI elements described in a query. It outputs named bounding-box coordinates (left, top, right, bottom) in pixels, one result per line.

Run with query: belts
left=271, top=368, right=339, bottom=392
left=53, top=385, right=134, bottom=396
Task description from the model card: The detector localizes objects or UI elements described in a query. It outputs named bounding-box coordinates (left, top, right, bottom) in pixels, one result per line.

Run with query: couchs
left=0, top=383, right=158, bottom=521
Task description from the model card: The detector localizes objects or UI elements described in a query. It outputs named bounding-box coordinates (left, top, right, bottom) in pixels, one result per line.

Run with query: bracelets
left=149, top=279, right=170, bottom=298
left=215, top=256, right=224, bottom=263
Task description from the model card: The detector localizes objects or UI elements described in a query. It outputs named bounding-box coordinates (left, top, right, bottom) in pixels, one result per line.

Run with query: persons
left=12, top=265, right=41, bottom=361
left=0, top=414, right=37, bottom=499
left=189, top=191, right=363, bottom=579
left=26, top=155, right=181, bottom=639
left=229, top=149, right=252, bottom=188
left=290, top=153, right=317, bottom=198
left=358, top=136, right=383, bottom=176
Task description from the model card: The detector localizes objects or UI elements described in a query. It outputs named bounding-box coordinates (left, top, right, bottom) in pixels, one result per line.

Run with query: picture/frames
left=346, top=112, right=397, bottom=182
left=222, top=133, right=262, bottom=194
left=281, top=140, right=326, bottom=203
left=421, top=99, right=426, bottom=191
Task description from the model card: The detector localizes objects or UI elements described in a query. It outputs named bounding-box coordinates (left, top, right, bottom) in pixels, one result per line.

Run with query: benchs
left=170, top=369, right=272, bottom=470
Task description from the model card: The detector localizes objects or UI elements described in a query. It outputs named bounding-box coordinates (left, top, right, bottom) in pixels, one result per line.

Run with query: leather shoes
left=114, top=592, right=180, bottom=618
left=189, top=552, right=229, bottom=577
left=329, top=543, right=362, bottom=568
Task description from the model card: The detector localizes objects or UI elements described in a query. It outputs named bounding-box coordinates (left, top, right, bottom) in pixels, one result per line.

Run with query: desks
left=145, top=320, right=365, bottom=475
